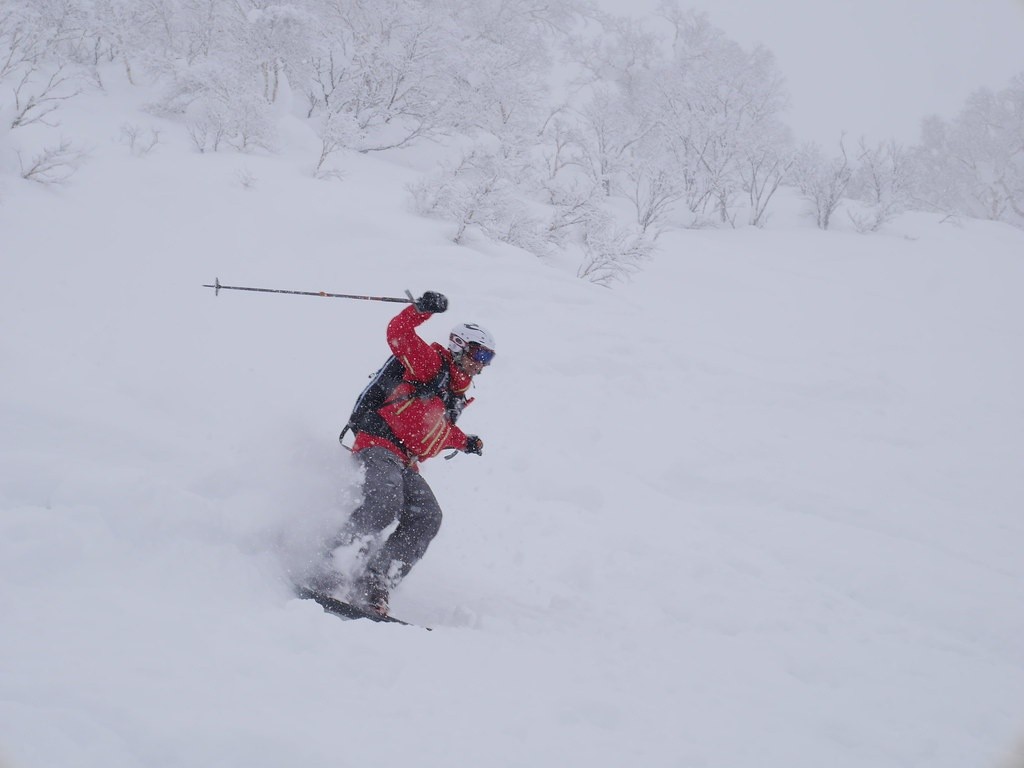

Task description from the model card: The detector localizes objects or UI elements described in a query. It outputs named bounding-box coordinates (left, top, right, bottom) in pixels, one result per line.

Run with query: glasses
left=467, top=343, right=496, bottom=367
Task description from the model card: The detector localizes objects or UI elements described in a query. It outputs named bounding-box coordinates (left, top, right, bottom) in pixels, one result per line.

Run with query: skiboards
left=294, top=579, right=436, bottom=631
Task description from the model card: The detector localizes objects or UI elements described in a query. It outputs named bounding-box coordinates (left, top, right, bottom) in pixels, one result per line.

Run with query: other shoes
left=345, top=570, right=388, bottom=617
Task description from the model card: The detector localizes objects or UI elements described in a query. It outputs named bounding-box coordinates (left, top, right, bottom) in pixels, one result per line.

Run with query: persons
left=306, top=290, right=498, bottom=616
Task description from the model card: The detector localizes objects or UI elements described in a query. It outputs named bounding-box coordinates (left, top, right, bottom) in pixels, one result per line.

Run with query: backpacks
left=339, top=348, right=465, bottom=458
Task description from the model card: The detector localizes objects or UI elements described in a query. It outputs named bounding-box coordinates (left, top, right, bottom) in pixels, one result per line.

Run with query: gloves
left=463, top=434, right=482, bottom=456
left=418, top=291, right=448, bottom=314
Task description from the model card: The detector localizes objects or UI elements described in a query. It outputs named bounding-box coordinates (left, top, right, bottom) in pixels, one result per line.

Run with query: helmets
left=447, top=322, right=497, bottom=356
